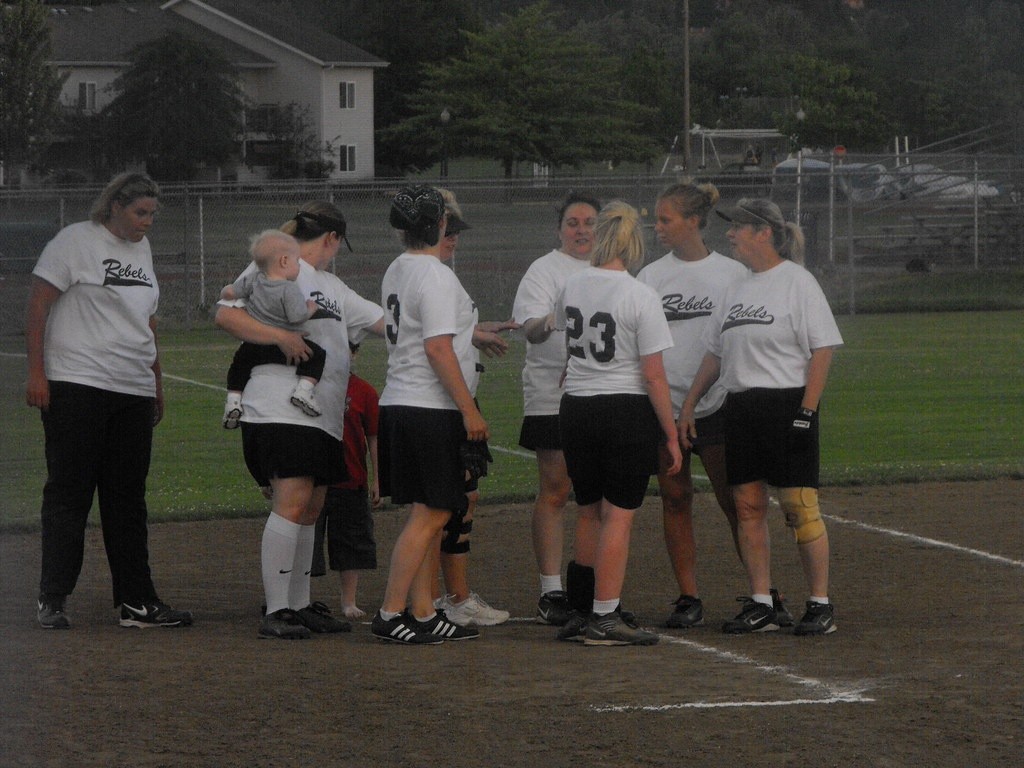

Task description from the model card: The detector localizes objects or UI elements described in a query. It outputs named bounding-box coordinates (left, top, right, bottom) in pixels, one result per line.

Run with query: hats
left=444, top=211, right=473, bottom=237
left=714, top=205, right=774, bottom=227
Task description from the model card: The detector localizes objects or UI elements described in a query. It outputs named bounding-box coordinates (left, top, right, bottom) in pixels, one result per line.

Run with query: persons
left=513, top=193, right=683, bottom=647
left=636, top=178, right=844, bottom=641
left=745, top=145, right=758, bottom=163
left=213, top=183, right=524, bottom=644
left=26, top=170, right=195, bottom=630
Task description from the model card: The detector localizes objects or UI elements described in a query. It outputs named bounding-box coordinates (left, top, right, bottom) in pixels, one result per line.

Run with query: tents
left=838, top=162, right=1002, bottom=266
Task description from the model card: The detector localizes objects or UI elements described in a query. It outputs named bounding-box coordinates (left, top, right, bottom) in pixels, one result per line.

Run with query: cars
left=710, top=163, right=772, bottom=193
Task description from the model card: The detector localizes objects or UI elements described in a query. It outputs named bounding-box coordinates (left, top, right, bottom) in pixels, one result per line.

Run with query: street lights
left=440, top=107, right=450, bottom=177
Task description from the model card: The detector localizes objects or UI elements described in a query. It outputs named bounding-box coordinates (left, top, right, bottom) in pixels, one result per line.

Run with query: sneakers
left=291, top=390, right=323, bottom=418
left=795, top=601, right=838, bottom=634
left=562, top=611, right=662, bottom=648
left=665, top=596, right=705, bottom=627
left=255, top=611, right=309, bottom=638
left=370, top=609, right=482, bottom=647
left=769, top=588, right=796, bottom=626
left=721, top=596, right=781, bottom=634
left=223, top=399, right=242, bottom=429
left=535, top=591, right=579, bottom=625
left=37, top=595, right=72, bottom=630
left=297, top=601, right=355, bottom=635
left=119, top=598, right=194, bottom=629
left=447, top=598, right=515, bottom=625
